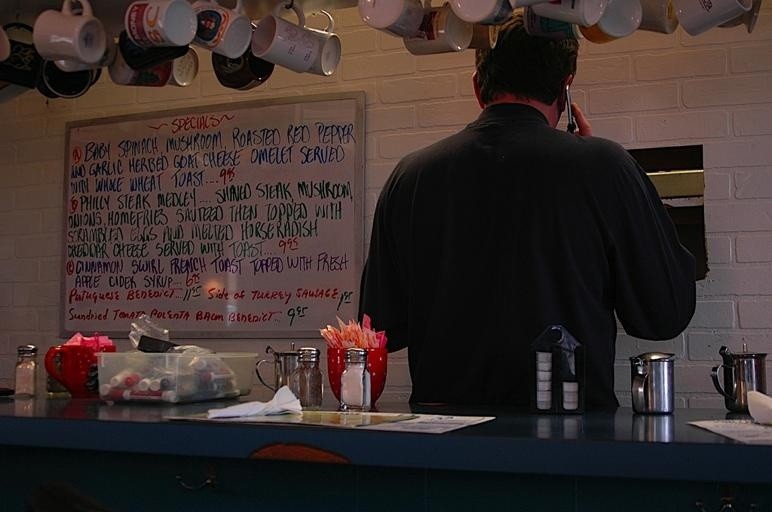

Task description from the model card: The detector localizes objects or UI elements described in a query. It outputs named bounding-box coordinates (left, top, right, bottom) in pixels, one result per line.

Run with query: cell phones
left=564, top=87, right=577, bottom=138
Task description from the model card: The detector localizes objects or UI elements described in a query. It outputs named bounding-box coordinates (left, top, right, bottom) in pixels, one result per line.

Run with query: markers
left=98, top=357, right=238, bottom=403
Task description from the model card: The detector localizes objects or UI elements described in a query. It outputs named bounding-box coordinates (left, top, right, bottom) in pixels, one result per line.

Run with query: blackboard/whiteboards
left=58, top=91, right=366, bottom=339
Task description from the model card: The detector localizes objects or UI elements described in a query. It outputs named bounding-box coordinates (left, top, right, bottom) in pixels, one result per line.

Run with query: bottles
left=14, top=344, right=38, bottom=399
left=292, top=347, right=327, bottom=412
left=340, top=348, right=371, bottom=413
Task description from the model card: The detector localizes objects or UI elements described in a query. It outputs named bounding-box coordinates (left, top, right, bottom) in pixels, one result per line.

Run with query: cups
left=357, top=0, right=766, bottom=57
left=0, top=0, right=341, bottom=98
left=44, top=343, right=116, bottom=404
left=326, top=347, right=388, bottom=410
left=710, top=346, right=768, bottom=416
left=629, top=351, right=675, bottom=416
left=252, top=345, right=321, bottom=409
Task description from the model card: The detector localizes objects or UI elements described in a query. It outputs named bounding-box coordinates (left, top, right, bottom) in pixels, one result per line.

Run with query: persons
left=359, top=9, right=698, bottom=407
